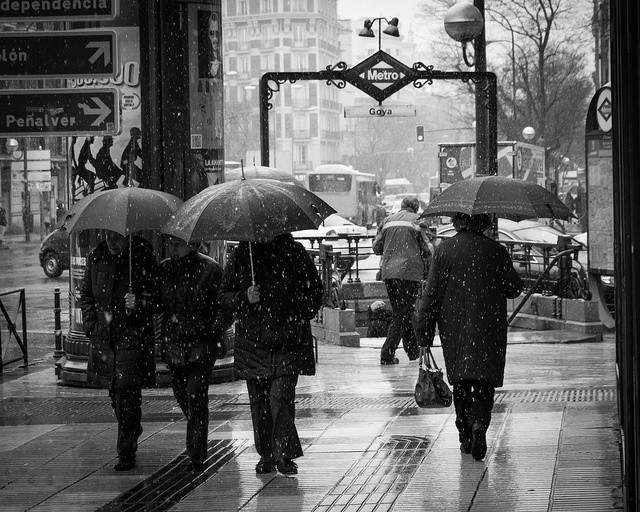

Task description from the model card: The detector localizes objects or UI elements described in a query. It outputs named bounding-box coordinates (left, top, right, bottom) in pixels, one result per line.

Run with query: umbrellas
left=223, top=156, right=306, bottom=189
left=417, top=173, right=579, bottom=241
left=53, top=185, right=185, bottom=316
left=157, top=159, right=338, bottom=286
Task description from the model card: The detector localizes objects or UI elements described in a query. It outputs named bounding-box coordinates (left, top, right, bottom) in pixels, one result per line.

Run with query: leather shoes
left=459, top=444, right=471, bottom=455
left=188, top=452, right=204, bottom=469
left=255, top=457, right=277, bottom=474
left=115, top=456, right=136, bottom=471
left=471, top=422, right=487, bottom=461
left=409, top=347, right=428, bottom=359
left=277, top=458, right=298, bottom=475
left=380, top=357, right=399, bottom=365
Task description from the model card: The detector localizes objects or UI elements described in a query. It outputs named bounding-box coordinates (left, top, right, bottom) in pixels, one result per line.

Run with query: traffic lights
left=416, top=125, right=425, bottom=142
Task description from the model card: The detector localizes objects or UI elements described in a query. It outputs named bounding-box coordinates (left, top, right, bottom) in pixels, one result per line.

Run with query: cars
left=389, top=201, right=424, bottom=217
left=430, top=217, right=614, bottom=318
left=381, top=195, right=399, bottom=212
left=291, top=212, right=371, bottom=270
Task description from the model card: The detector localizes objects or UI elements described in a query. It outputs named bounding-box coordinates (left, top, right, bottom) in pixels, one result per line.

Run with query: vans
left=40, top=215, right=69, bottom=277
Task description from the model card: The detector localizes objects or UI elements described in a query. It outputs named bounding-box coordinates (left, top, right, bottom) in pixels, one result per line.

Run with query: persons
left=217, top=233, right=324, bottom=474
left=80, top=230, right=158, bottom=471
left=418, top=213, right=524, bottom=460
left=157, top=235, right=236, bottom=468
left=372, top=196, right=435, bottom=365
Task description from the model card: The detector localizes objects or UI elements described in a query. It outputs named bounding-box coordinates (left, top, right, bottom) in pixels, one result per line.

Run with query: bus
left=307, top=164, right=381, bottom=229
left=383, top=177, right=415, bottom=194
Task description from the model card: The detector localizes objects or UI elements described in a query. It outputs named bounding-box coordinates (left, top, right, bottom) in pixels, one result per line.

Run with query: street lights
left=359, top=16, right=400, bottom=51
left=4, top=137, right=33, bottom=243
left=471, top=120, right=477, bottom=134
left=443, top=2, right=488, bottom=177
left=521, top=125, right=536, bottom=143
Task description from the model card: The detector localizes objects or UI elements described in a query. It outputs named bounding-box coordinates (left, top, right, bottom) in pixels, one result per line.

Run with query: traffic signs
left=0, top=0, right=120, bottom=22
left=0, top=29, right=120, bottom=81
left=0, top=88, right=121, bottom=137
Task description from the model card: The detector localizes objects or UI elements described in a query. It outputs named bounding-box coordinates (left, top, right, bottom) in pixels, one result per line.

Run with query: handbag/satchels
left=415, top=368, right=452, bottom=409
